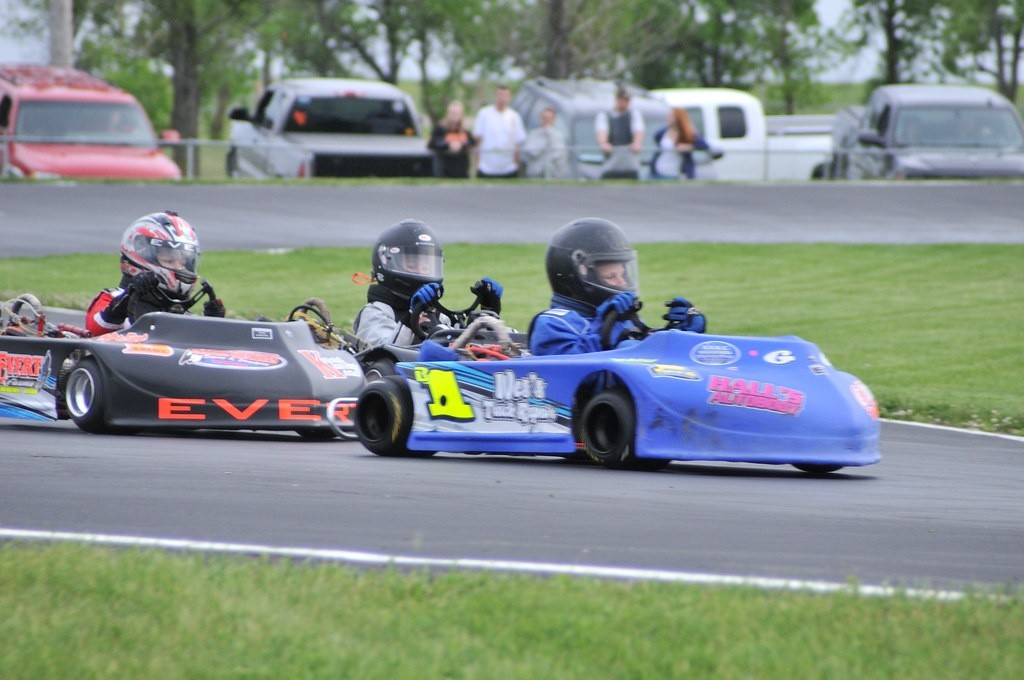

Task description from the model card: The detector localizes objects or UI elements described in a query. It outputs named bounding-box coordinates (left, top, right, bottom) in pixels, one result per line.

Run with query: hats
left=618, top=89, right=629, bottom=100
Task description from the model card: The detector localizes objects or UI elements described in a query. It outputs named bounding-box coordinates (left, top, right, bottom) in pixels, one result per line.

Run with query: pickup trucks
left=834, top=84, right=1024, bottom=178
left=648, top=87, right=834, bottom=179
left=219, top=77, right=437, bottom=177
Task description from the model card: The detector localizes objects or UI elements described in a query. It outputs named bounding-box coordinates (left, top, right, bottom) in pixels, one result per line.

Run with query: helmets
left=118, top=210, right=201, bottom=304
left=370, top=222, right=445, bottom=302
left=546, top=221, right=639, bottom=313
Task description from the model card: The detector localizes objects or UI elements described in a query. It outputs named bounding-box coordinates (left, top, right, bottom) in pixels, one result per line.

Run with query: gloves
left=203, top=299, right=225, bottom=319
left=595, top=289, right=636, bottom=318
left=410, top=282, right=441, bottom=311
left=130, top=271, right=159, bottom=296
left=665, top=300, right=708, bottom=333
left=471, top=278, right=504, bottom=310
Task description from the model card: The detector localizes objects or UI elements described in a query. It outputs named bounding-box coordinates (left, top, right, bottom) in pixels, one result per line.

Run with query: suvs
left=1, top=64, right=183, bottom=180
left=513, top=76, right=671, bottom=178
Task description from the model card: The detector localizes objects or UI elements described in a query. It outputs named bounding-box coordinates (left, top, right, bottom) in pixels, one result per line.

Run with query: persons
left=596, top=85, right=644, bottom=179
left=85, top=213, right=227, bottom=337
left=528, top=217, right=706, bottom=356
left=354, top=219, right=504, bottom=348
left=471, top=84, right=526, bottom=178
left=519, top=107, right=568, bottom=179
left=651, top=106, right=714, bottom=181
left=427, top=100, right=476, bottom=178
